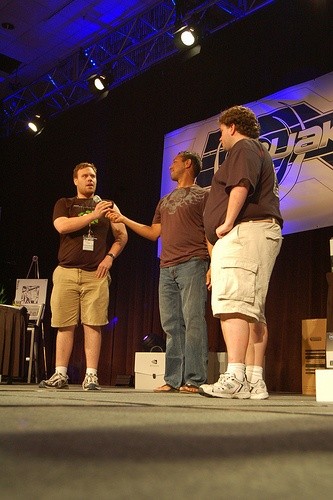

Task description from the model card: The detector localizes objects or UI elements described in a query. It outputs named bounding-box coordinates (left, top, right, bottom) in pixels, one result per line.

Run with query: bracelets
left=107, top=253, right=115, bottom=260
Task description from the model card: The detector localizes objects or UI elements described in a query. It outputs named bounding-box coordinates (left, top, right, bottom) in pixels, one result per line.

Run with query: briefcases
left=135, top=346, right=166, bottom=390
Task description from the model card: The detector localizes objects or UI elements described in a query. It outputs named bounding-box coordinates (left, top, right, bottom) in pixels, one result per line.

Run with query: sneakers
left=38, top=370, right=69, bottom=390
left=198, top=373, right=251, bottom=399
left=246, top=379, right=269, bottom=399
left=82, top=373, right=101, bottom=391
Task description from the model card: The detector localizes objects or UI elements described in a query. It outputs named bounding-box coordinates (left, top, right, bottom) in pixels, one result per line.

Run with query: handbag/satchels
left=14, top=260, right=48, bottom=320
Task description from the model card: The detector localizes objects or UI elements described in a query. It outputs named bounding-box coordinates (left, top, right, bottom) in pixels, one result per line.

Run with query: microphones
left=92, top=195, right=102, bottom=204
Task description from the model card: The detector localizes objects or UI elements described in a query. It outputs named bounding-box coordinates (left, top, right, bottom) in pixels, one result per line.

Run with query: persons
left=104, top=150, right=215, bottom=393
left=199, top=105, right=284, bottom=401
left=39, top=162, right=128, bottom=391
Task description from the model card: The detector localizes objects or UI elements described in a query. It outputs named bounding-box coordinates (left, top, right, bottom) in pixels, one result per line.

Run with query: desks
left=0, top=304, right=31, bottom=385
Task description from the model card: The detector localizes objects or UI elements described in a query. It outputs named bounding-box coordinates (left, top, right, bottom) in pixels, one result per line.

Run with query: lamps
left=17, top=114, right=47, bottom=148
left=172, top=25, right=201, bottom=60
left=86, top=73, right=110, bottom=101
left=141, top=333, right=166, bottom=352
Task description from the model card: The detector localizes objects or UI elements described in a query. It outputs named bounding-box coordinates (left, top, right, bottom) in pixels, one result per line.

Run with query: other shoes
left=180, top=384, right=199, bottom=394
left=154, top=384, right=179, bottom=393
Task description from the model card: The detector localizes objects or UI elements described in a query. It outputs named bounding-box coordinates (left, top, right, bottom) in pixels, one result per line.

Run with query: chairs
left=12, top=302, right=45, bottom=384
